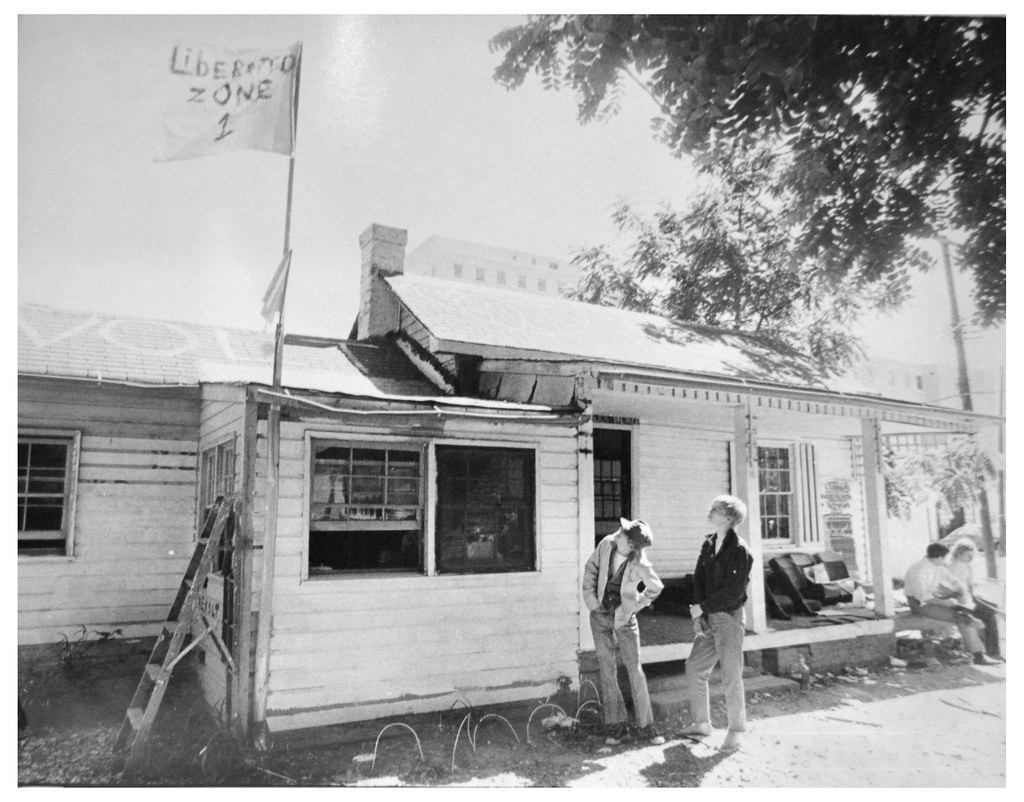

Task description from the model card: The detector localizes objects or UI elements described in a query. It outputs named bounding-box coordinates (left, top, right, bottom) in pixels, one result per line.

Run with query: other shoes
left=965, top=616, right=986, bottom=630
left=973, top=653, right=1004, bottom=665
left=676, top=722, right=714, bottom=736
left=719, top=729, right=744, bottom=754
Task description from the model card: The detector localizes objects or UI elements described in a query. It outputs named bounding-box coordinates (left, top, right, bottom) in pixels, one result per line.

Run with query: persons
left=579, top=515, right=665, bottom=740
left=900, top=541, right=1001, bottom=666
left=673, top=496, right=756, bottom=755
left=944, top=535, right=1005, bottom=665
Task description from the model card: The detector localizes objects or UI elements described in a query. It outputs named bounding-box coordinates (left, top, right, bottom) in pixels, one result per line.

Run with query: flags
left=148, top=39, right=304, bottom=165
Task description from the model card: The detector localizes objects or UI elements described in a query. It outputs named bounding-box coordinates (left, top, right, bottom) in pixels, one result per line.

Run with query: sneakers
left=604, top=723, right=631, bottom=746
left=646, top=724, right=666, bottom=745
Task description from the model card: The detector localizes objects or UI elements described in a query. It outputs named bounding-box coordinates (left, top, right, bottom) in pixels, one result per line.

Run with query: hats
left=620, top=517, right=653, bottom=549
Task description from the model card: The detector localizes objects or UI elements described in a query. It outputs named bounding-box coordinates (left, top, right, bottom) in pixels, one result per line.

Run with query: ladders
left=112, top=497, right=233, bottom=781
